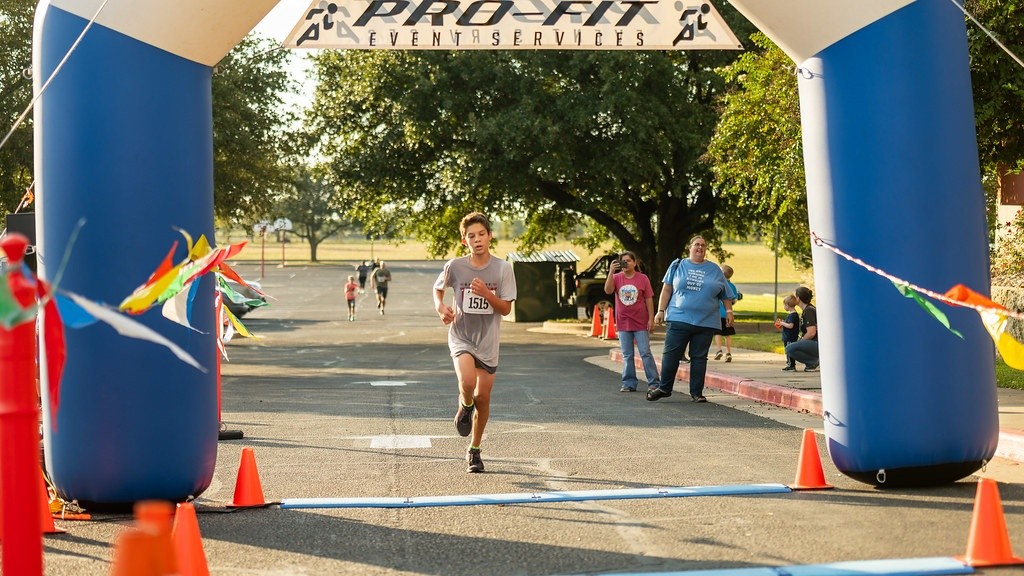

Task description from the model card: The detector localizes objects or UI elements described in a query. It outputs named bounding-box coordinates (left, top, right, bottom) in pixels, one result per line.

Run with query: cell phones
left=615, top=261, right=627, bottom=268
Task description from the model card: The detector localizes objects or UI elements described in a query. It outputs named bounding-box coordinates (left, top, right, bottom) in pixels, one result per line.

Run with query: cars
left=214, top=271, right=266, bottom=330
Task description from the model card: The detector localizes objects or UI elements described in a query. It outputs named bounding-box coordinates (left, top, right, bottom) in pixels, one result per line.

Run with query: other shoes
left=804, top=361, right=820, bottom=371
left=648, top=386, right=657, bottom=391
left=646, top=389, right=671, bottom=401
left=381, top=310, right=384, bottom=315
left=725, top=353, right=732, bottom=362
left=620, top=384, right=636, bottom=392
left=714, top=350, right=723, bottom=360
left=679, top=355, right=689, bottom=363
left=691, top=395, right=706, bottom=402
left=377, top=301, right=380, bottom=307
left=781, top=367, right=796, bottom=372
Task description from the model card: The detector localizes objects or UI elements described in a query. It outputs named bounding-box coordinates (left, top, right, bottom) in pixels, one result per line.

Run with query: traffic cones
left=228, top=450, right=271, bottom=508
left=790, top=426, right=833, bottom=491
left=117, top=502, right=208, bottom=576
left=965, top=478, right=1023, bottom=566
left=601, top=308, right=620, bottom=340
left=40, top=470, right=61, bottom=532
left=589, top=305, right=602, bottom=337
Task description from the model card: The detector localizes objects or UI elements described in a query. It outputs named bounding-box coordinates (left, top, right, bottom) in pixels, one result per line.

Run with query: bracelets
left=659, top=310, right=664, bottom=312
left=726, top=309, right=732, bottom=312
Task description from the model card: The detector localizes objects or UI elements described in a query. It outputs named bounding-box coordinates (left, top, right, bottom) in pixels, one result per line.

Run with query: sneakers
left=465, top=449, right=485, bottom=472
left=454, top=394, right=474, bottom=437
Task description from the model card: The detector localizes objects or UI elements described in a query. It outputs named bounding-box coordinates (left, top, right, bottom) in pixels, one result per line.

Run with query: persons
left=371, top=257, right=380, bottom=271
left=344, top=276, right=359, bottom=321
left=785, top=286, right=819, bottom=372
left=356, top=260, right=368, bottom=294
left=432, top=212, right=517, bottom=473
left=645, top=236, right=736, bottom=403
left=780, top=295, right=800, bottom=372
left=714, top=266, right=738, bottom=362
left=370, top=260, right=391, bottom=315
left=604, top=252, right=660, bottom=392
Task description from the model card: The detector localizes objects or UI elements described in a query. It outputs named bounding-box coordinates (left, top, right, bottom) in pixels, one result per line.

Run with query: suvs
left=575, top=254, right=648, bottom=321
